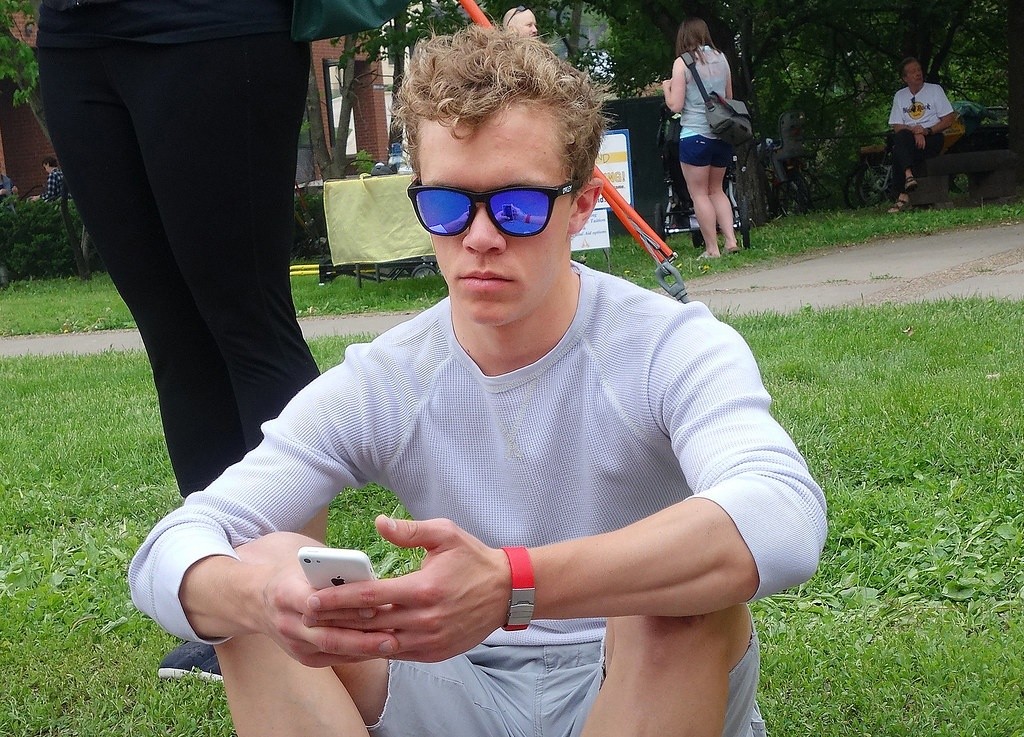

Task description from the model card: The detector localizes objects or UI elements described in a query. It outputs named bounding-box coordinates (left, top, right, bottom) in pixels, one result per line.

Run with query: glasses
left=911, top=97, right=916, bottom=113
left=507, top=3, right=534, bottom=27
left=408, top=174, right=581, bottom=238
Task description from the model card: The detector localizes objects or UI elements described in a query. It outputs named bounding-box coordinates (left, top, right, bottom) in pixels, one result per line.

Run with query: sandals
left=886, top=199, right=913, bottom=213
left=904, top=177, right=919, bottom=193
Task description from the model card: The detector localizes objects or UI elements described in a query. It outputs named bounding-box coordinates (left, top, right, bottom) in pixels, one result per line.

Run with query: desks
left=322, top=172, right=439, bottom=282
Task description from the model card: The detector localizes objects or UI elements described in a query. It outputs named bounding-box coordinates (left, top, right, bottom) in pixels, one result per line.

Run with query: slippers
left=719, top=243, right=739, bottom=253
left=697, top=251, right=721, bottom=259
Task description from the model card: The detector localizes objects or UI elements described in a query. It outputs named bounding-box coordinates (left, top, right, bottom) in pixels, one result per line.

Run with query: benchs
left=911, top=149, right=1020, bottom=208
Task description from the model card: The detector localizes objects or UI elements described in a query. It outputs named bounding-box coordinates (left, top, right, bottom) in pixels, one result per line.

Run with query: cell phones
left=298, top=547, right=376, bottom=590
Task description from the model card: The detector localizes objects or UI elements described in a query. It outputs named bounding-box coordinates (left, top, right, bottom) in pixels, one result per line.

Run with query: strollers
left=655, top=104, right=752, bottom=248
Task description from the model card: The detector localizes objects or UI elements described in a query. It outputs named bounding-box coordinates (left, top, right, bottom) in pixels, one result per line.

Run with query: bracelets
left=927, top=128, right=932, bottom=136
left=501, top=547, right=535, bottom=631
left=525, top=214, right=531, bottom=223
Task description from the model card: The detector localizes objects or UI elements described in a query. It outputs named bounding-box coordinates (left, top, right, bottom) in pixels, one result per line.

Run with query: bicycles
left=757, top=138, right=809, bottom=219
left=844, top=107, right=1007, bottom=210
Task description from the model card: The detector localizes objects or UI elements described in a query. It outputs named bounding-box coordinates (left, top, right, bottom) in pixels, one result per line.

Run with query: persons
left=495, top=203, right=546, bottom=226
left=0, top=163, right=18, bottom=203
left=888, top=58, right=955, bottom=213
left=33, top=1, right=321, bottom=679
left=503, top=7, right=537, bottom=35
left=32, top=157, right=62, bottom=202
left=129, top=26, right=827, bottom=737
left=429, top=211, right=469, bottom=234
left=662, top=18, right=739, bottom=260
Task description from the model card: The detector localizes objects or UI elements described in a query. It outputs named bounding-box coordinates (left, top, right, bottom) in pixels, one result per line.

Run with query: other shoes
left=195, top=655, right=224, bottom=682
left=157, top=642, right=216, bottom=678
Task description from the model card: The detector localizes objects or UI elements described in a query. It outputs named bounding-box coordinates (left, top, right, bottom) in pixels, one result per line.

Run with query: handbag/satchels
left=291, top=0, right=417, bottom=43
left=704, top=91, right=753, bottom=146
